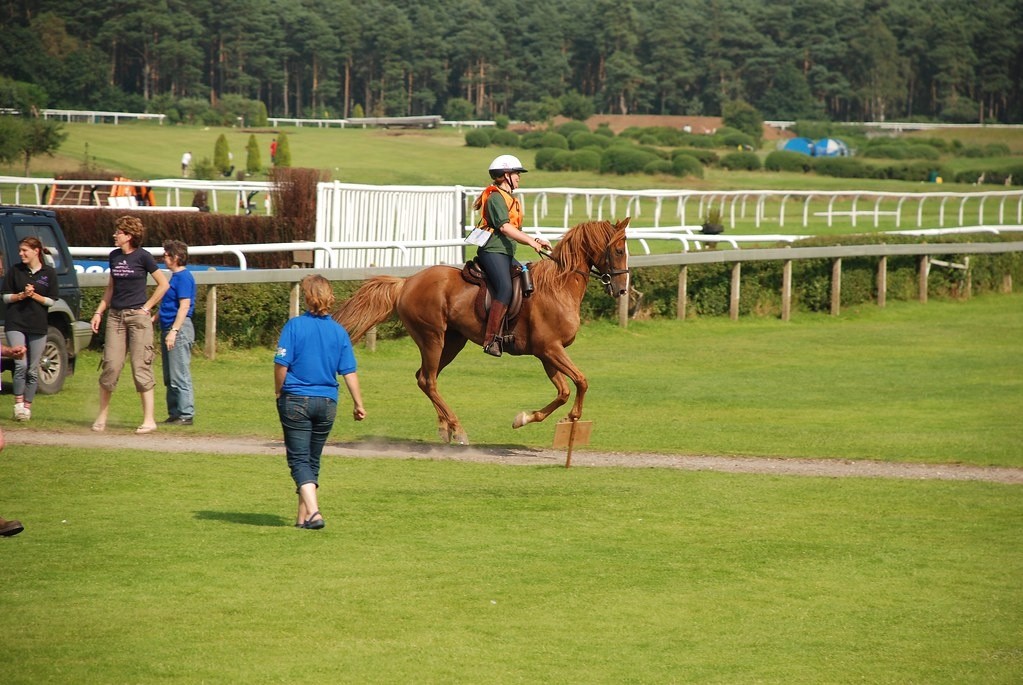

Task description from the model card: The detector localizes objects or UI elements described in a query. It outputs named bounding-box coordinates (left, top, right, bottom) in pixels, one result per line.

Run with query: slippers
left=91, top=421, right=105, bottom=431
left=134, top=426, right=157, bottom=434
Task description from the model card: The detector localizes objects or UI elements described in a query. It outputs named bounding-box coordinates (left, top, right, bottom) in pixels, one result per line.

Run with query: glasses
left=116, top=230, right=129, bottom=235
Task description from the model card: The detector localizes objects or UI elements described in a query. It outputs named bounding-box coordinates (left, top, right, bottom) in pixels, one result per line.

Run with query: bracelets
left=534, top=238, right=540, bottom=242
left=17, top=292, right=25, bottom=300
left=141, top=306, right=149, bottom=312
left=93, top=312, right=102, bottom=319
left=171, top=327, right=179, bottom=333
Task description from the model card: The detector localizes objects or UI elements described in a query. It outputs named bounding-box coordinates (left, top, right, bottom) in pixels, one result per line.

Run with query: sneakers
left=14, top=402, right=25, bottom=420
left=21, top=407, right=31, bottom=421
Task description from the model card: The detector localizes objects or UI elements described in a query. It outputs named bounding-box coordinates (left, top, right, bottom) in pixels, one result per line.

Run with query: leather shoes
left=0, top=517, right=24, bottom=536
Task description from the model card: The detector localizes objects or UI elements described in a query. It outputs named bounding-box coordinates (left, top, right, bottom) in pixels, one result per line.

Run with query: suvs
left=0, top=207, right=80, bottom=395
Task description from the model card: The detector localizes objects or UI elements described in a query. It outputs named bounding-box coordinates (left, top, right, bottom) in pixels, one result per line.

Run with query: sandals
left=295, top=511, right=325, bottom=530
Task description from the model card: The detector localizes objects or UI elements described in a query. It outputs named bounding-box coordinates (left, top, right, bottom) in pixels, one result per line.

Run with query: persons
left=181, top=152, right=192, bottom=177
left=0, top=236, right=60, bottom=421
left=151, top=240, right=195, bottom=426
left=474, top=155, right=551, bottom=357
left=192, top=190, right=209, bottom=212
left=929, top=167, right=941, bottom=182
left=91, top=215, right=170, bottom=433
left=0, top=344, right=27, bottom=536
left=270, top=138, right=278, bottom=163
left=273, top=274, right=367, bottom=530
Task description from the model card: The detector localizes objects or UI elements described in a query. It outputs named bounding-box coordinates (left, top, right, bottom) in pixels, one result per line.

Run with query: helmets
left=489, top=155, right=528, bottom=178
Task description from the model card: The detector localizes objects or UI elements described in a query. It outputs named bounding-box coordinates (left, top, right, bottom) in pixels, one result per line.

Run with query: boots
left=484, top=298, right=507, bottom=356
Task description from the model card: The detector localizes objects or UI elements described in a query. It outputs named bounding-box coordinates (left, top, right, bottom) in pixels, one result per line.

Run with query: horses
left=332, top=214, right=631, bottom=445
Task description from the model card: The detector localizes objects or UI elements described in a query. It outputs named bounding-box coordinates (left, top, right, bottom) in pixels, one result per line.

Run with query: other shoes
left=157, top=416, right=193, bottom=425
